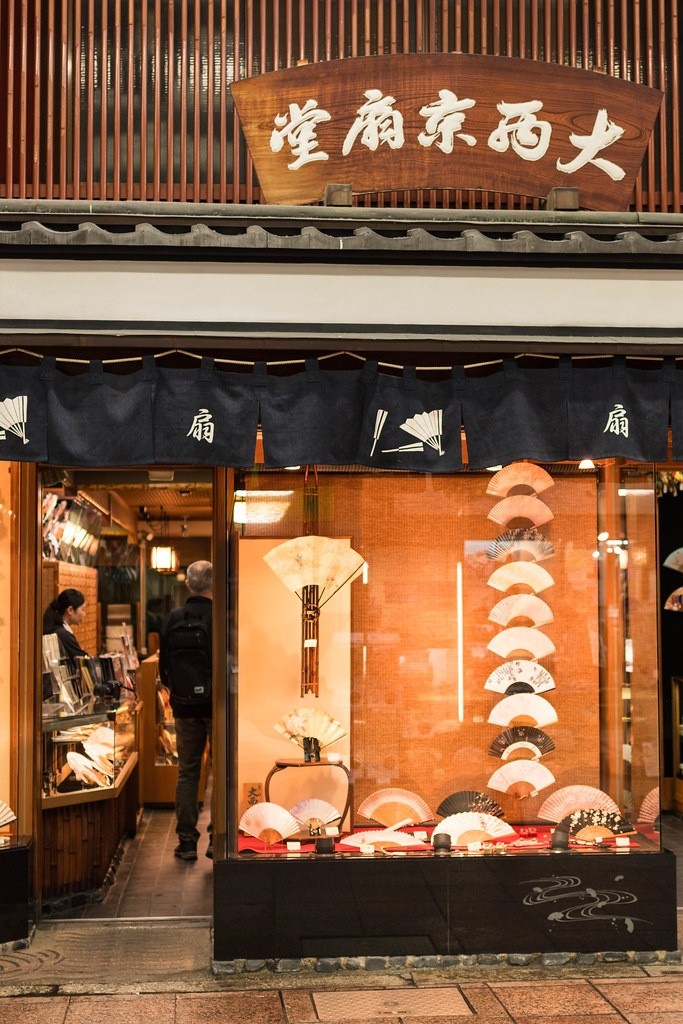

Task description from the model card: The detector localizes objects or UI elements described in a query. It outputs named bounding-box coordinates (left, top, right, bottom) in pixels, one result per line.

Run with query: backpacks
left=165, top=619, right=212, bottom=706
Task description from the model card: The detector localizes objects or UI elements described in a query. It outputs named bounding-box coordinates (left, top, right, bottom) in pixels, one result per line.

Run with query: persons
left=157, top=559, right=213, bottom=862
left=42, top=588, right=91, bottom=697
left=145, top=594, right=176, bottom=635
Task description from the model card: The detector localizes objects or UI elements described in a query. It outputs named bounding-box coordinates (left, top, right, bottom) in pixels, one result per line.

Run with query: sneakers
left=175, top=842, right=197, bottom=860
left=205, top=842, right=213, bottom=858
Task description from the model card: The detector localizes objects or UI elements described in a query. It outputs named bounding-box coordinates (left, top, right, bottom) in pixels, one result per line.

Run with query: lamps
left=151, top=505, right=180, bottom=573
